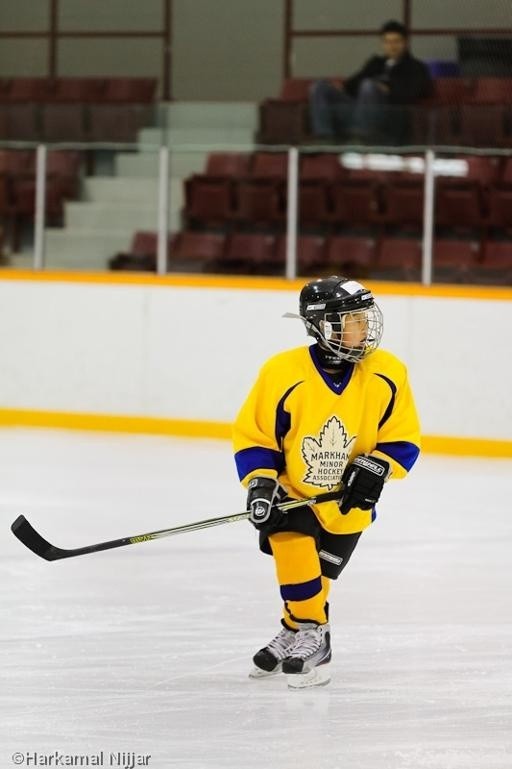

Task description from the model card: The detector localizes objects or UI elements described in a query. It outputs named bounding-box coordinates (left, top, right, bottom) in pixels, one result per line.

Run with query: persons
left=305, top=17, right=432, bottom=157
left=229, top=273, right=425, bottom=674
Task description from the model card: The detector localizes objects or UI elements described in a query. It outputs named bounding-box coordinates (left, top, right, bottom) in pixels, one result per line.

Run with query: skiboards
left=11, top=491, right=347, bottom=562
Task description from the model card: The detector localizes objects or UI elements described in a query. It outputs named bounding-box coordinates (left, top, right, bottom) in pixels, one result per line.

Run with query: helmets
left=301, top=276, right=385, bottom=364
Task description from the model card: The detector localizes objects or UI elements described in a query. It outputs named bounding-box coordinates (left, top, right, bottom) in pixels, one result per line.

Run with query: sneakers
left=254, top=626, right=298, bottom=671
left=282, top=622, right=331, bottom=673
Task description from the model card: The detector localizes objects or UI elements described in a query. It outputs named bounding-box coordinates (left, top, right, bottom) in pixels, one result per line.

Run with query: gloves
left=340, top=455, right=389, bottom=514
left=246, top=477, right=289, bottom=533
